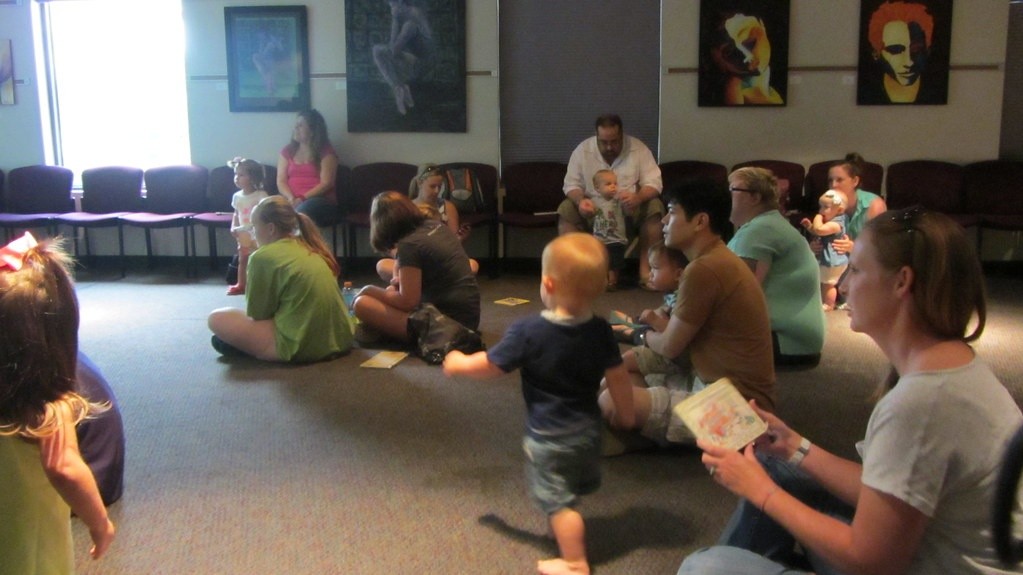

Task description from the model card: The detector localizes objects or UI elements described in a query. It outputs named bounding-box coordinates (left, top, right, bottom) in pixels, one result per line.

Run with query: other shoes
left=212, top=334, right=244, bottom=358
left=352, top=322, right=383, bottom=343
left=227, top=284, right=245, bottom=295
left=607, top=282, right=616, bottom=291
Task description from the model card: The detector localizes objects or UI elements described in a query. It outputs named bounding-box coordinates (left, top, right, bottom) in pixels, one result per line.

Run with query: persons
left=810, top=163, right=886, bottom=310
left=350, top=162, right=481, bottom=345
left=621, top=240, right=691, bottom=376
left=226, top=158, right=268, bottom=295
left=76, top=353, right=125, bottom=506
left=801, top=189, right=848, bottom=311
left=0, top=231, right=115, bottom=575
left=276, top=110, right=339, bottom=225
left=676, top=210, right=1023, bottom=575
left=559, top=117, right=667, bottom=292
left=207, top=196, right=353, bottom=362
left=442, top=233, right=634, bottom=575
left=610, top=167, right=823, bottom=368
left=598, top=178, right=777, bottom=452
left=592, top=170, right=639, bottom=289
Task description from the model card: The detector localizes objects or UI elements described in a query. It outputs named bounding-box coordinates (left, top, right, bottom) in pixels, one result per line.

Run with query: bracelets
left=296, top=194, right=306, bottom=202
left=787, top=437, right=811, bottom=470
left=761, top=487, right=783, bottom=513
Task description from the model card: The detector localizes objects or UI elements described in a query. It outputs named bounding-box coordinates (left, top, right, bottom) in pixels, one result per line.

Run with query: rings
left=710, top=466, right=716, bottom=476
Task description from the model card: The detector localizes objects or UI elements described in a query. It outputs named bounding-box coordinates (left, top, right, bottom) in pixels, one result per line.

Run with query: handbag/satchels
left=407, top=304, right=486, bottom=365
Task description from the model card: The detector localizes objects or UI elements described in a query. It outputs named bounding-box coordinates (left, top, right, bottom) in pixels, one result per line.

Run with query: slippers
left=609, top=320, right=649, bottom=344
left=611, top=308, right=632, bottom=326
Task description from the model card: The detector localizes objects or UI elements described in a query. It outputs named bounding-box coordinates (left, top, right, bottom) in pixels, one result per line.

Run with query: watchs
left=640, top=328, right=655, bottom=346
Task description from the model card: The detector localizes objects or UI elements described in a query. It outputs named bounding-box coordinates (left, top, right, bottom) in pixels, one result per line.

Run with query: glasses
left=419, top=166, right=437, bottom=180
left=729, top=184, right=766, bottom=200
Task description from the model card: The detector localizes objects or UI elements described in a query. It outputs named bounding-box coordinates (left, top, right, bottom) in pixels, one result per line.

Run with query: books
left=673, top=378, right=768, bottom=451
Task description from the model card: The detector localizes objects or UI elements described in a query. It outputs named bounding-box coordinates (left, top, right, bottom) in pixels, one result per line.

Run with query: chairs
left=0, top=160, right=1023, bottom=276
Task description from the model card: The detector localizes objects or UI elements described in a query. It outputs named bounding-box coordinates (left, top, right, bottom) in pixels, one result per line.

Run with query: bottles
left=342, top=282, right=354, bottom=316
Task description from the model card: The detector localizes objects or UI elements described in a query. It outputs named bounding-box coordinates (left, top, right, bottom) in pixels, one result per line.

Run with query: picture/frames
left=224, top=5, right=311, bottom=112
left=344, top=0, right=468, bottom=133
